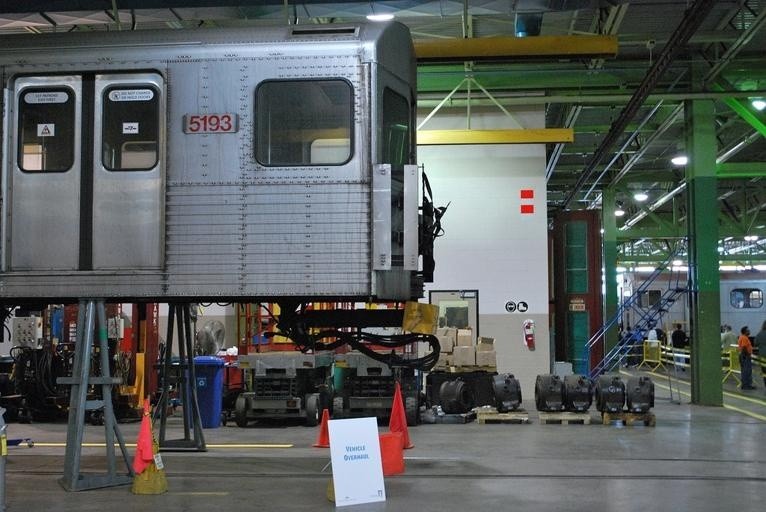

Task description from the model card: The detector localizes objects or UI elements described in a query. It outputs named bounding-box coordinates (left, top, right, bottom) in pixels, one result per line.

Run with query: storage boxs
left=425, top=326, right=497, bottom=374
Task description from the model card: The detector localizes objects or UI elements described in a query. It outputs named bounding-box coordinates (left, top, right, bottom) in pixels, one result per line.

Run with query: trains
left=0, top=19, right=436, bottom=302
left=623, top=272, right=761, bottom=342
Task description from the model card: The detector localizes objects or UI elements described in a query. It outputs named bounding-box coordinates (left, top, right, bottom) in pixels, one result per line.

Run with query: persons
left=737, top=326, right=756, bottom=390
left=618, top=322, right=736, bottom=372
left=754, top=320, right=766, bottom=387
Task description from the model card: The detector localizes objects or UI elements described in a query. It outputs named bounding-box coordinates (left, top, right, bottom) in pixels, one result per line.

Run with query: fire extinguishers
left=525, top=323, right=535, bottom=348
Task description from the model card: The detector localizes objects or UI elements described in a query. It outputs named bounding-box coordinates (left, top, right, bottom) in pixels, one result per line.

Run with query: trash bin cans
left=185, top=356, right=225, bottom=428
